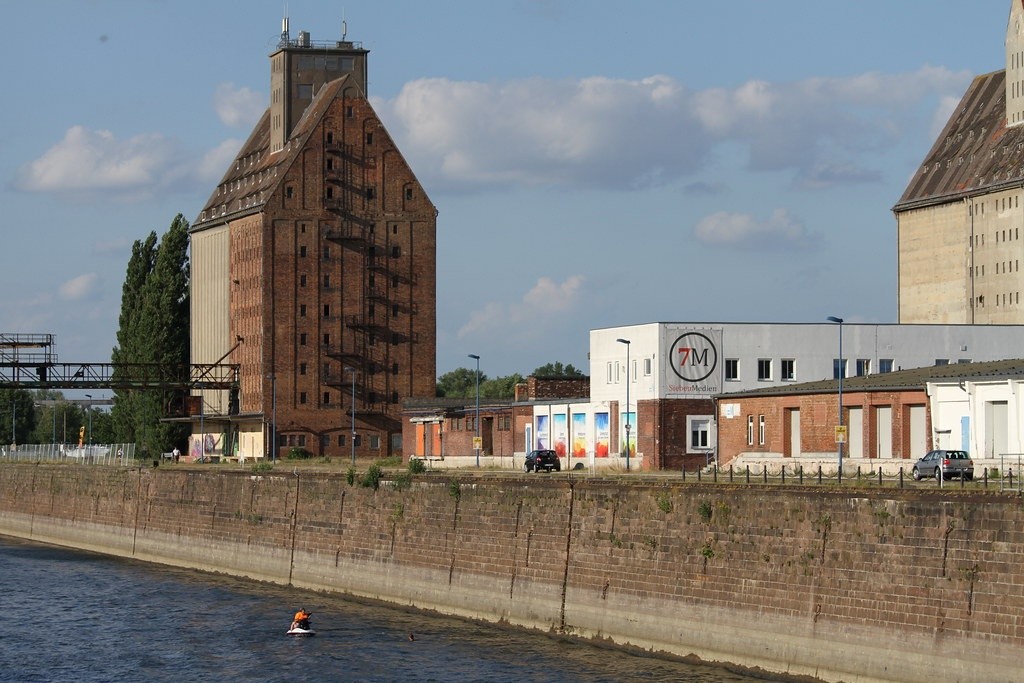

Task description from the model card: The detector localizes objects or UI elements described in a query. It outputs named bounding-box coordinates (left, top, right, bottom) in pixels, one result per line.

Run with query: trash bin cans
left=153, top=460, right=159, bottom=467
left=989, top=468, right=998, bottom=478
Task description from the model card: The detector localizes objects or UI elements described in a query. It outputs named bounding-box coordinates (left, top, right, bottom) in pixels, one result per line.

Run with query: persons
left=291, top=608, right=308, bottom=630
left=408, top=634, right=413, bottom=640
left=172, top=447, right=181, bottom=464
left=118, top=448, right=122, bottom=458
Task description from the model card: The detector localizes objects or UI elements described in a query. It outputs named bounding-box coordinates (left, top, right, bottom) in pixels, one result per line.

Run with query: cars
left=912, top=450, right=974, bottom=480
left=524, top=450, right=560, bottom=473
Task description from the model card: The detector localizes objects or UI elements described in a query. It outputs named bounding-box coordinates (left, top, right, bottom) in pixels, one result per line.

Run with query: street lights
left=468, top=354, right=480, bottom=468
left=616, top=339, right=631, bottom=474
left=266, top=376, right=276, bottom=465
left=827, top=316, right=844, bottom=483
left=194, top=384, right=207, bottom=464
left=49, top=394, right=92, bottom=446
left=9, top=401, right=15, bottom=444
left=345, top=367, right=355, bottom=467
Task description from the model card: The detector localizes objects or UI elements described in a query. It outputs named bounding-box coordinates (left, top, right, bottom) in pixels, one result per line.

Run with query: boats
left=287, top=628, right=317, bottom=637
